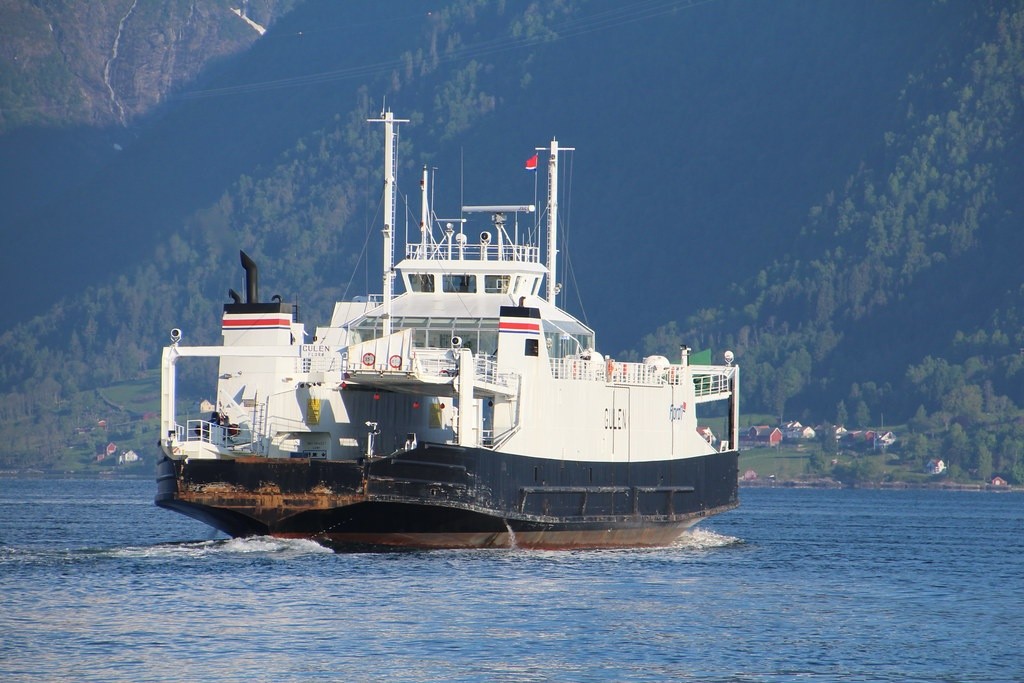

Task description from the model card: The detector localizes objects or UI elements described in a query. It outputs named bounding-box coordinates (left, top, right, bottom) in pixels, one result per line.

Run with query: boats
left=154, top=101, right=743, bottom=544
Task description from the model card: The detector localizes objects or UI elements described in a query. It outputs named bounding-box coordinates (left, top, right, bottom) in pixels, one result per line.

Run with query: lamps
left=480, top=231, right=492, bottom=244
left=724, top=351, right=734, bottom=364
left=170, top=328, right=182, bottom=346
left=456, top=233, right=468, bottom=247
left=451, top=336, right=462, bottom=351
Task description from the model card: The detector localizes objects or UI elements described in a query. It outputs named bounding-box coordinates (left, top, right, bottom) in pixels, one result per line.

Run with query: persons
left=208, top=412, right=219, bottom=425
left=405, top=437, right=411, bottom=452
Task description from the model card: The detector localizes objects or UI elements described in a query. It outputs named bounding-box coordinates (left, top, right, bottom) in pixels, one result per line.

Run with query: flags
left=525, top=155, right=538, bottom=170
